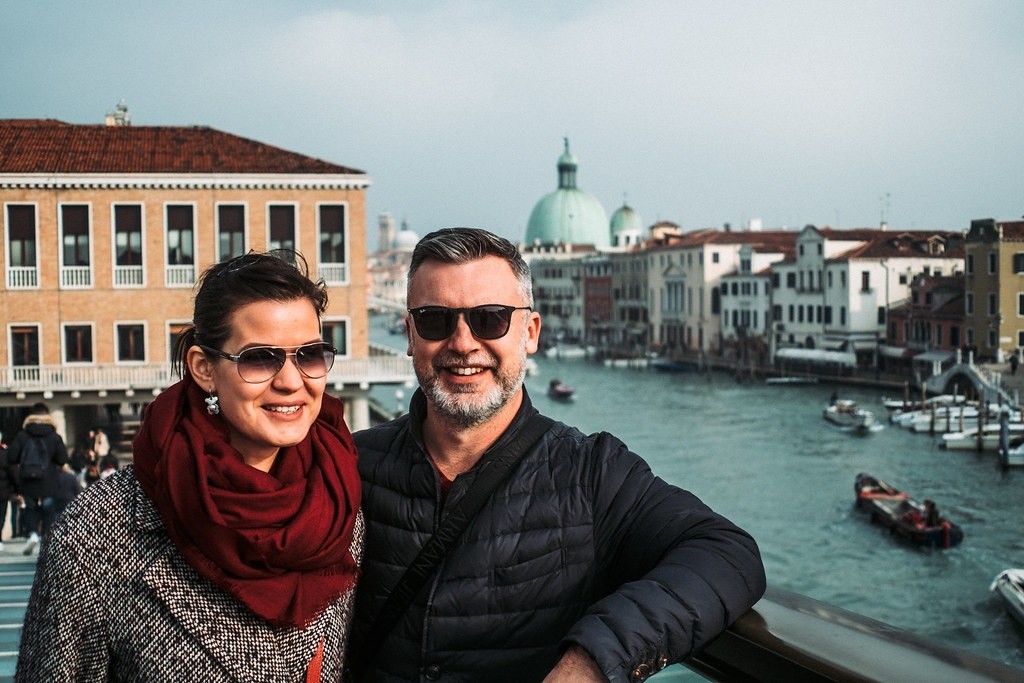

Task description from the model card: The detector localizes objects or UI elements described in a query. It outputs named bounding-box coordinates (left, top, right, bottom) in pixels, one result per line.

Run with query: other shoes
left=23, top=535, right=38, bottom=556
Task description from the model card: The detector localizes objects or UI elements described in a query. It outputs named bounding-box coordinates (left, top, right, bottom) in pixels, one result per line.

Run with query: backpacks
left=19, top=435, right=54, bottom=491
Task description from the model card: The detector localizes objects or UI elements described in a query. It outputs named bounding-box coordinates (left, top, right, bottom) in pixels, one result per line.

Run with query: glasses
left=408, top=304, right=532, bottom=341
left=199, top=342, right=338, bottom=384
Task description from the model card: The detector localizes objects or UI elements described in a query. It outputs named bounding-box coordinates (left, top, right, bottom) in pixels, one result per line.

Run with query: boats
left=839, top=422, right=883, bottom=437
left=547, top=379, right=575, bottom=402
left=824, top=398, right=874, bottom=428
left=859, top=471, right=963, bottom=549
left=884, top=392, right=1024, bottom=468
left=988, top=569, right=1024, bottom=631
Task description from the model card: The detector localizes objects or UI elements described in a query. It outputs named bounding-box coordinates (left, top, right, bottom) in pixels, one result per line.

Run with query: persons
left=42, top=429, right=119, bottom=528
left=923, top=499, right=939, bottom=526
left=11, top=498, right=24, bottom=538
left=6, top=402, right=67, bottom=555
left=12, top=253, right=364, bottom=683
left=343, top=228, right=766, bottom=683
left=0, top=432, right=9, bottom=542
